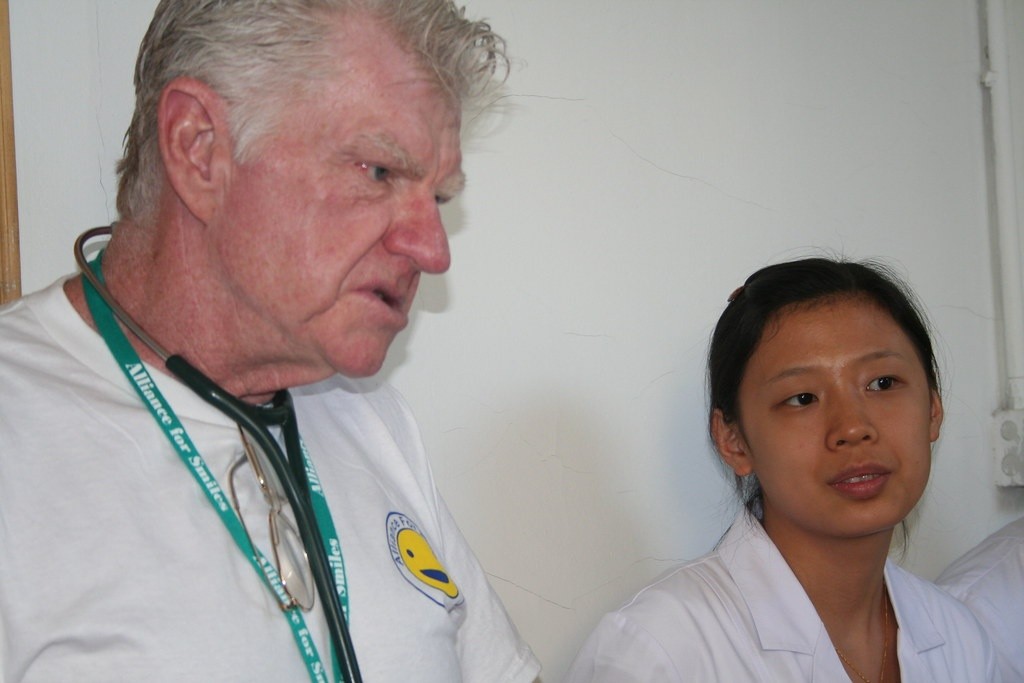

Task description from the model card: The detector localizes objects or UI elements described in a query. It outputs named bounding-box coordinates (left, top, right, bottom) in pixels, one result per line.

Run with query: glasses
left=229, top=422, right=316, bottom=613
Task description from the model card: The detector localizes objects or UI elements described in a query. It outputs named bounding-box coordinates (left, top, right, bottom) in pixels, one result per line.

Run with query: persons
left=0, top=0, right=548, bottom=683
left=560, top=259, right=1024, bottom=683
left=933, top=514, right=1024, bottom=683
